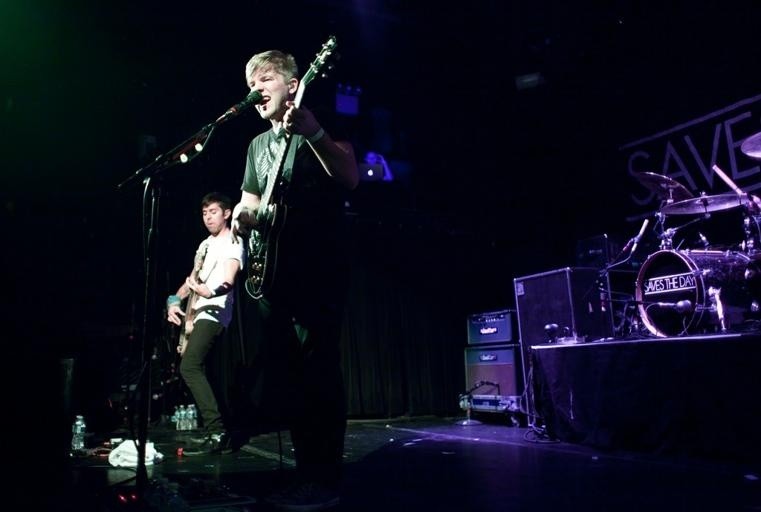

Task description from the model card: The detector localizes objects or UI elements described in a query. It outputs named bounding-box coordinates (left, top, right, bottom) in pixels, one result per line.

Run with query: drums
left=635, top=249, right=761, bottom=338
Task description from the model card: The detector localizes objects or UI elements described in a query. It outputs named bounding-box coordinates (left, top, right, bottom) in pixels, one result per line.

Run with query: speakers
left=511, top=266, right=615, bottom=426
left=464, top=344, right=521, bottom=401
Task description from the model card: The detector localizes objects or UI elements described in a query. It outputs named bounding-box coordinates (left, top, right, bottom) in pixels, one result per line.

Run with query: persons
left=167, top=191, right=248, bottom=459
left=229, top=49, right=362, bottom=510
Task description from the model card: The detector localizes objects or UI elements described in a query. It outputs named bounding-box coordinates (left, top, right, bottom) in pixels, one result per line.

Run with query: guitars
left=245, top=36, right=339, bottom=295
left=177, top=242, right=208, bottom=358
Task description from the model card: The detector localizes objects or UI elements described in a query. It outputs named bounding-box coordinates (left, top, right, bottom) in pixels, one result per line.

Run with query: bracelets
left=210, top=281, right=233, bottom=299
left=166, top=293, right=182, bottom=308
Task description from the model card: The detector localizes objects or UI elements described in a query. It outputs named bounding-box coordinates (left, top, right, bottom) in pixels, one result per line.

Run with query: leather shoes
left=183, top=430, right=234, bottom=456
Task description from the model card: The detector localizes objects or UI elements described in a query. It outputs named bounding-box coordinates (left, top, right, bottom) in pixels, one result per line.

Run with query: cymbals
left=659, top=193, right=748, bottom=214
left=741, top=132, right=761, bottom=158
left=637, top=171, right=694, bottom=203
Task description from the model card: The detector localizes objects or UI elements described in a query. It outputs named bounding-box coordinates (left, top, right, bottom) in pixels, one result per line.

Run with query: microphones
left=675, top=298, right=692, bottom=316
left=634, top=216, right=650, bottom=243
left=216, top=86, right=263, bottom=127
left=480, top=379, right=496, bottom=387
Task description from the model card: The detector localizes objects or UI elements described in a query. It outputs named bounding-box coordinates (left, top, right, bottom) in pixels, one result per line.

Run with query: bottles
left=70, top=414, right=87, bottom=453
left=172, top=403, right=199, bottom=430
